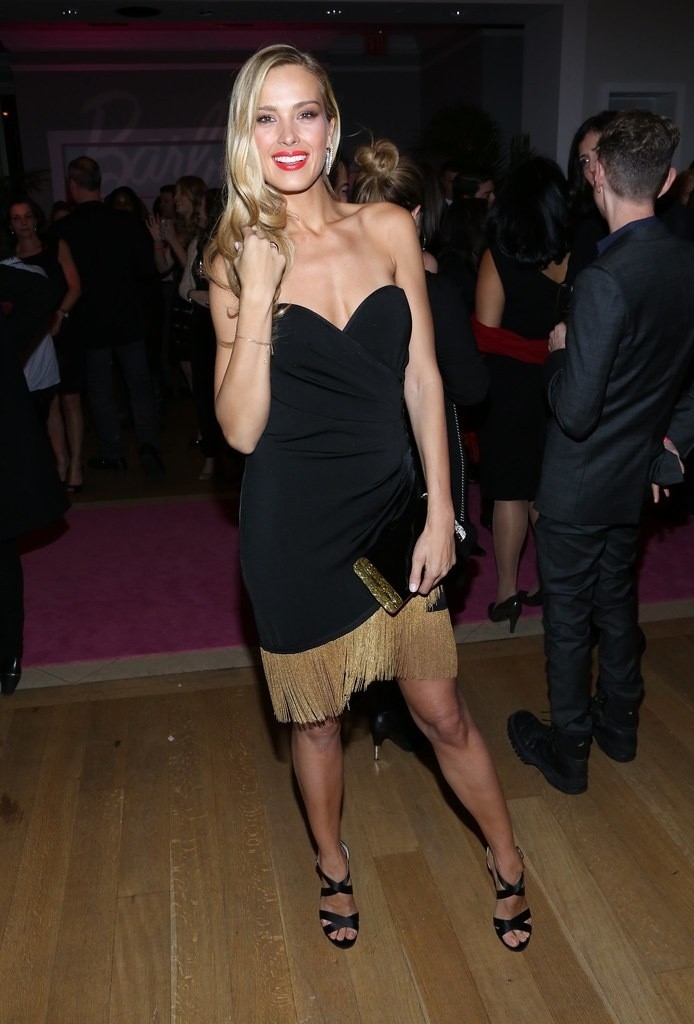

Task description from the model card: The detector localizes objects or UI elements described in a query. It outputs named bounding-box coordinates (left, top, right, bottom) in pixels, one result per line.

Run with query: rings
left=270, top=242, right=278, bottom=252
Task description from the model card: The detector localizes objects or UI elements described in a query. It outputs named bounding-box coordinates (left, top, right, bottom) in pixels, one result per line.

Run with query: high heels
left=518, top=588, right=543, bottom=608
left=369, top=709, right=428, bottom=761
left=483, top=846, right=532, bottom=953
left=316, top=841, right=360, bottom=949
left=66, top=463, right=85, bottom=493
left=488, top=594, right=522, bottom=634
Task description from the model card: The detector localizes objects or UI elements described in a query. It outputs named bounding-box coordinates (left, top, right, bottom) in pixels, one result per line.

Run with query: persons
left=207, top=45, right=531, bottom=953
left=0, top=110, right=694, bottom=793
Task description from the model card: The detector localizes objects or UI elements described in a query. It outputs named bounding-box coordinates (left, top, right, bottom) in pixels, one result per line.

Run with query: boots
left=506, top=709, right=589, bottom=796
left=591, top=696, right=638, bottom=763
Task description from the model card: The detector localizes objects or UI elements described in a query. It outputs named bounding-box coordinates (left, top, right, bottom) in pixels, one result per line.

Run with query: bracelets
left=58, top=309, right=69, bottom=318
left=186, top=290, right=194, bottom=302
left=234, top=336, right=271, bottom=363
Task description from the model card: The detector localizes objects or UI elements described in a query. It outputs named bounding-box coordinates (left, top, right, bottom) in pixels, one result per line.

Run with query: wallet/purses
left=352, top=491, right=466, bottom=617
